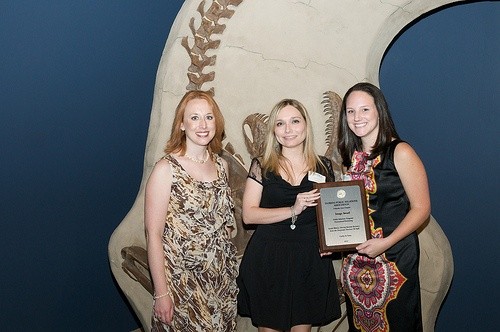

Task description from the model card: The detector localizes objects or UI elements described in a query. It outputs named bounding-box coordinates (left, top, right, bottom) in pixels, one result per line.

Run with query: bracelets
left=287, top=203, right=299, bottom=229
left=153, top=291, right=173, bottom=300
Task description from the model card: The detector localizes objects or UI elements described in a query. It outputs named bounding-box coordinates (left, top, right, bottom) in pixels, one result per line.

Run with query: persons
left=237, top=97, right=342, bottom=332
left=333, top=82, right=431, bottom=332
left=142, top=90, right=241, bottom=332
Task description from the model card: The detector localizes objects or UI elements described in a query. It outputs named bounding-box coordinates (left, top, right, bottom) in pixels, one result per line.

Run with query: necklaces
left=180, top=148, right=210, bottom=163
